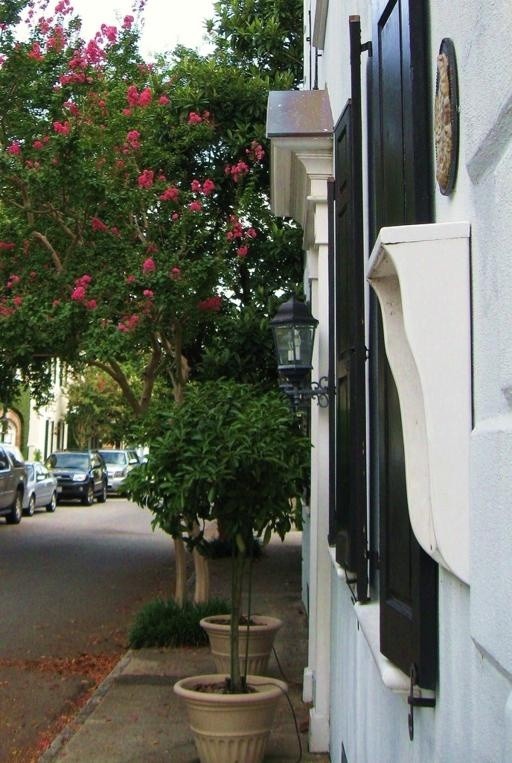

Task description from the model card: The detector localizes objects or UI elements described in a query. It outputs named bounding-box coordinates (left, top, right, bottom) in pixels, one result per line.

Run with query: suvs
left=0, top=442, right=141, bottom=522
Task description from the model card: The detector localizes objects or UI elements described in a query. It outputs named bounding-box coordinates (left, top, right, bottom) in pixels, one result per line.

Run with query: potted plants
left=115, top=380, right=315, bottom=763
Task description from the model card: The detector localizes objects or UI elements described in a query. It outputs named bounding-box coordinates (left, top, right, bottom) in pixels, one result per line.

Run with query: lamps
left=270, top=290, right=331, bottom=409
